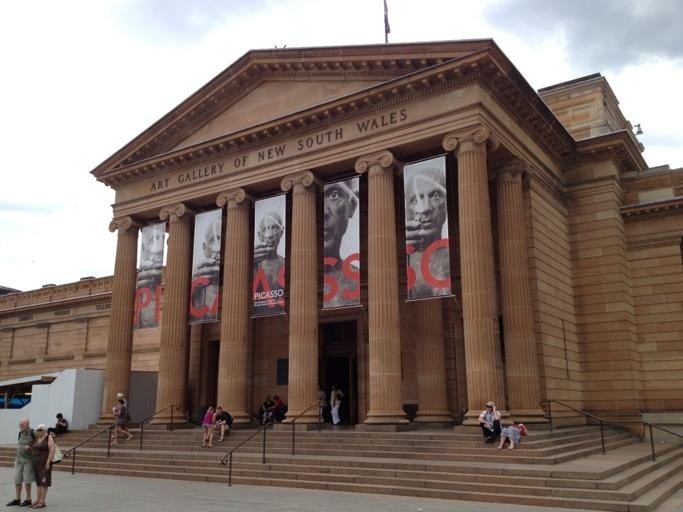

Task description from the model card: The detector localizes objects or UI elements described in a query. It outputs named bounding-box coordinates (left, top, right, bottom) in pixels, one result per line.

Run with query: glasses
left=35, top=429, right=47, bottom=432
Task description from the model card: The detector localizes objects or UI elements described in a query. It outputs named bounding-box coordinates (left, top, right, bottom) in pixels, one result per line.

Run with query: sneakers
left=19, top=499, right=33, bottom=507
left=5, top=499, right=21, bottom=507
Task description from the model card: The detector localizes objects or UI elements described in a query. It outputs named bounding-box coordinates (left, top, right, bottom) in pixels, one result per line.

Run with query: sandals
left=28, top=501, right=46, bottom=509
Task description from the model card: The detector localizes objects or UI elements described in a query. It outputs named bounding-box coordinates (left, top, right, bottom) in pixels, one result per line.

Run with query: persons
left=330, top=384, right=345, bottom=425
left=317, top=385, right=326, bottom=423
left=29, top=424, right=55, bottom=508
left=48, top=413, right=67, bottom=436
left=479, top=401, right=501, bottom=443
left=111, top=392, right=127, bottom=439
left=136, top=225, right=164, bottom=329
left=254, top=211, right=286, bottom=315
left=202, top=394, right=284, bottom=447
left=6, top=418, right=35, bottom=506
left=405, top=168, right=451, bottom=300
left=111, top=399, right=133, bottom=444
left=497, top=423, right=529, bottom=450
left=190, top=217, right=221, bottom=319
left=322, top=179, right=359, bottom=308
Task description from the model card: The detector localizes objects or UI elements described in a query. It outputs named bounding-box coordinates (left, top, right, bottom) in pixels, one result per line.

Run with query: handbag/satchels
left=46, top=434, right=63, bottom=465
